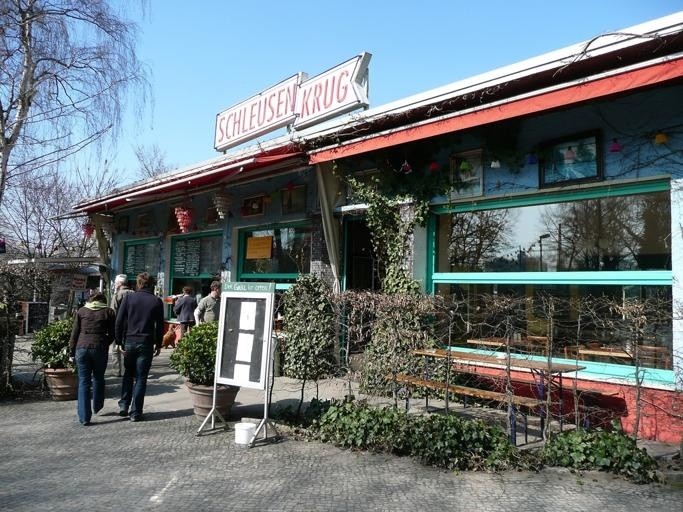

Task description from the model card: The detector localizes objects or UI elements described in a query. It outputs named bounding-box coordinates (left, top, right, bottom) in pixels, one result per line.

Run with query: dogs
left=161, top=324, right=178, bottom=349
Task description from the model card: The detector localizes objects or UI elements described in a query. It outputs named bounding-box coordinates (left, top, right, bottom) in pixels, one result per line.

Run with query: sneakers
left=119, top=410, right=144, bottom=422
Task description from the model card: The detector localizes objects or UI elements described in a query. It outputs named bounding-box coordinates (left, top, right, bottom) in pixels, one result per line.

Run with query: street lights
left=539, top=234, right=551, bottom=272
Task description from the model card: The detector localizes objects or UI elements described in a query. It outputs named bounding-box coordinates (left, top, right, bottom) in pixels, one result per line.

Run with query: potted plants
left=170, top=320, right=241, bottom=421
left=25, top=315, right=81, bottom=400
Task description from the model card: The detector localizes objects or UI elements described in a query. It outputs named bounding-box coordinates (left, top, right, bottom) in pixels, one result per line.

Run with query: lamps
left=175, top=182, right=234, bottom=234
left=426, top=129, right=670, bottom=173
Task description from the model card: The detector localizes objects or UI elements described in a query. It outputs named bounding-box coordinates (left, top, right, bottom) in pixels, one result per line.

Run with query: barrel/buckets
left=235, top=422, right=256, bottom=444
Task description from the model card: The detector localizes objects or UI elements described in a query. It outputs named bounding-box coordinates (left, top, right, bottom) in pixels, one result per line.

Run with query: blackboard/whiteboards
left=215, top=293, right=272, bottom=391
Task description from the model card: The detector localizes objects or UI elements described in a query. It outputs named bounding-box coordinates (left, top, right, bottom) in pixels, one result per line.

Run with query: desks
left=467, top=336, right=528, bottom=351
left=408, top=341, right=584, bottom=439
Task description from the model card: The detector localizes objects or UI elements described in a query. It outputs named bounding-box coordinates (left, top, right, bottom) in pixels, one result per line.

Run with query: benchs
left=384, top=371, right=546, bottom=448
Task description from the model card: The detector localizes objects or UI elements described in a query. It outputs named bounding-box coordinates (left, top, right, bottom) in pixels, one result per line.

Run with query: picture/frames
left=240, top=192, right=267, bottom=219
left=447, top=145, right=484, bottom=202
left=538, top=130, right=605, bottom=190
left=277, top=183, right=308, bottom=217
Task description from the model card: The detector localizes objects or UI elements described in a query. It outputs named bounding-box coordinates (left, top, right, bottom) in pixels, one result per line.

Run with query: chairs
left=527, top=334, right=553, bottom=355
left=562, top=341, right=669, bottom=370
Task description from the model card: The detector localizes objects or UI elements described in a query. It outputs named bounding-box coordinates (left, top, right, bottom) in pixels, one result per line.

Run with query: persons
left=171, top=284, right=197, bottom=339
left=67, top=290, right=115, bottom=424
left=112, top=271, right=163, bottom=424
left=108, top=274, right=134, bottom=377
left=192, top=280, right=222, bottom=327
